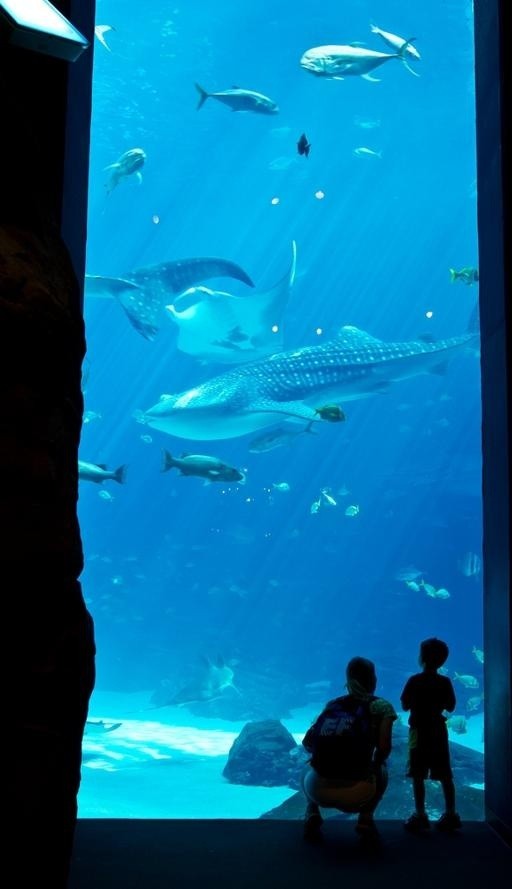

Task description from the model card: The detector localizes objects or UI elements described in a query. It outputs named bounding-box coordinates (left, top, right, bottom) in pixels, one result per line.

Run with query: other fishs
left=354, top=147, right=384, bottom=159
left=252, top=426, right=320, bottom=451
left=310, top=404, right=346, bottom=423
left=84, top=257, right=255, bottom=338
left=449, top=269, right=478, bottom=287
left=86, top=367, right=154, bottom=446
left=136, top=299, right=478, bottom=440
left=194, top=82, right=280, bottom=114
left=169, top=239, right=299, bottom=366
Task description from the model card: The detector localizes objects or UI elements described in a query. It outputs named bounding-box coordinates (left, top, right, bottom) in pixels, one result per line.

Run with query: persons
left=302, top=656, right=398, bottom=839
left=400, top=638, right=462, bottom=831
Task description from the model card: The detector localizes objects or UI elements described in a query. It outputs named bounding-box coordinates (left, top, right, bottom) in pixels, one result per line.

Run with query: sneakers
left=356, top=817, right=385, bottom=835
left=302, top=806, right=325, bottom=829
left=435, top=812, right=463, bottom=831
left=401, top=811, right=433, bottom=829
